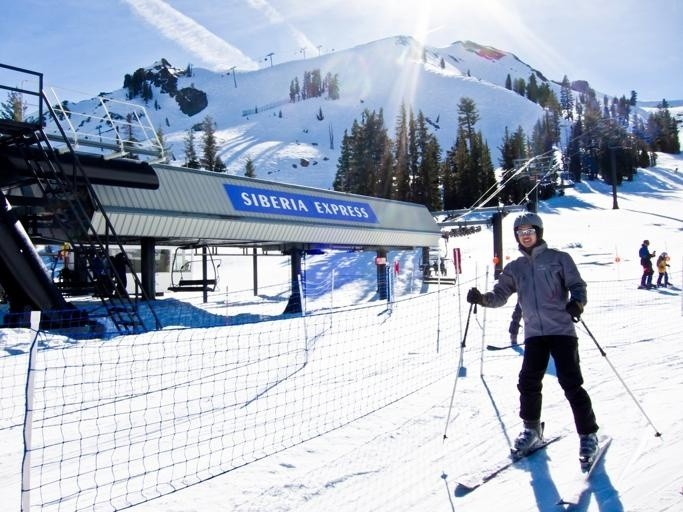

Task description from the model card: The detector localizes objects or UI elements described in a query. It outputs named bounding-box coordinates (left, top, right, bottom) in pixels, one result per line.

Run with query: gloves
left=566, top=300, right=583, bottom=318
left=467, top=289, right=482, bottom=304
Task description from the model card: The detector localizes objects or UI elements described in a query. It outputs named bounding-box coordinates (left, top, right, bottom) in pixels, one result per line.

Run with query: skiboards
left=487, top=343, right=525, bottom=350
left=454, top=434, right=613, bottom=505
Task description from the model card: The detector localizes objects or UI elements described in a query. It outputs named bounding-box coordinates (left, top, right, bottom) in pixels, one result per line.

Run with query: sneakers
left=514, top=430, right=540, bottom=449
left=579, top=432, right=598, bottom=457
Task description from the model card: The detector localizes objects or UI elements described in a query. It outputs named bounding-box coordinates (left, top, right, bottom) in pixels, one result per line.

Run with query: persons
left=393, top=259, right=400, bottom=276
left=639, top=240, right=656, bottom=287
left=439, top=260, right=447, bottom=276
left=657, top=251, right=672, bottom=286
left=46, top=242, right=128, bottom=297
left=432, top=261, right=438, bottom=275
left=465, top=212, right=602, bottom=457
left=422, top=259, right=432, bottom=276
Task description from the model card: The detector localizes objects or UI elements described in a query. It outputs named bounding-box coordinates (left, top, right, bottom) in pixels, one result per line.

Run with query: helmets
left=514, top=214, right=543, bottom=245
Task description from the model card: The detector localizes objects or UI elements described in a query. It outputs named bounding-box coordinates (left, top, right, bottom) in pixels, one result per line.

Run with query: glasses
left=515, top=229, right=536, bottom=236
